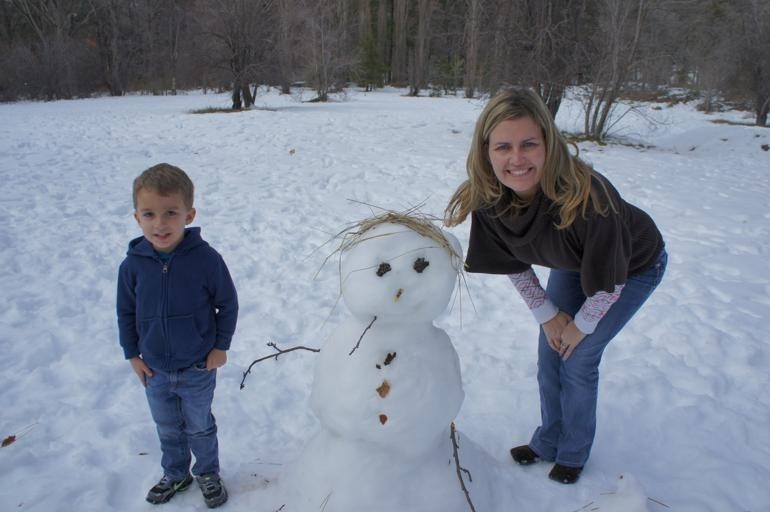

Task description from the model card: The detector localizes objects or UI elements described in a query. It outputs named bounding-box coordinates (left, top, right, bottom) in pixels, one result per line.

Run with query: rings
left=560, top=343, right=567, bottom=350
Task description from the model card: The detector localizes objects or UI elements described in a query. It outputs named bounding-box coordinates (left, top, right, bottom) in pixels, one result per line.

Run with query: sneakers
left=146, top=471, right=194, bottom=504
left=511, top=445, right=539, bottom=465
left=548, top=463, right=584, bottom=484
left=196, top=472, right=228, bottom=508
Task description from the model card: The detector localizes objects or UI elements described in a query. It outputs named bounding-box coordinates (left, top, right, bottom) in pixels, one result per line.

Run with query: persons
left=116, top=163, right=238, bottom=507
left=444, top=88, right=668, bottom=484
